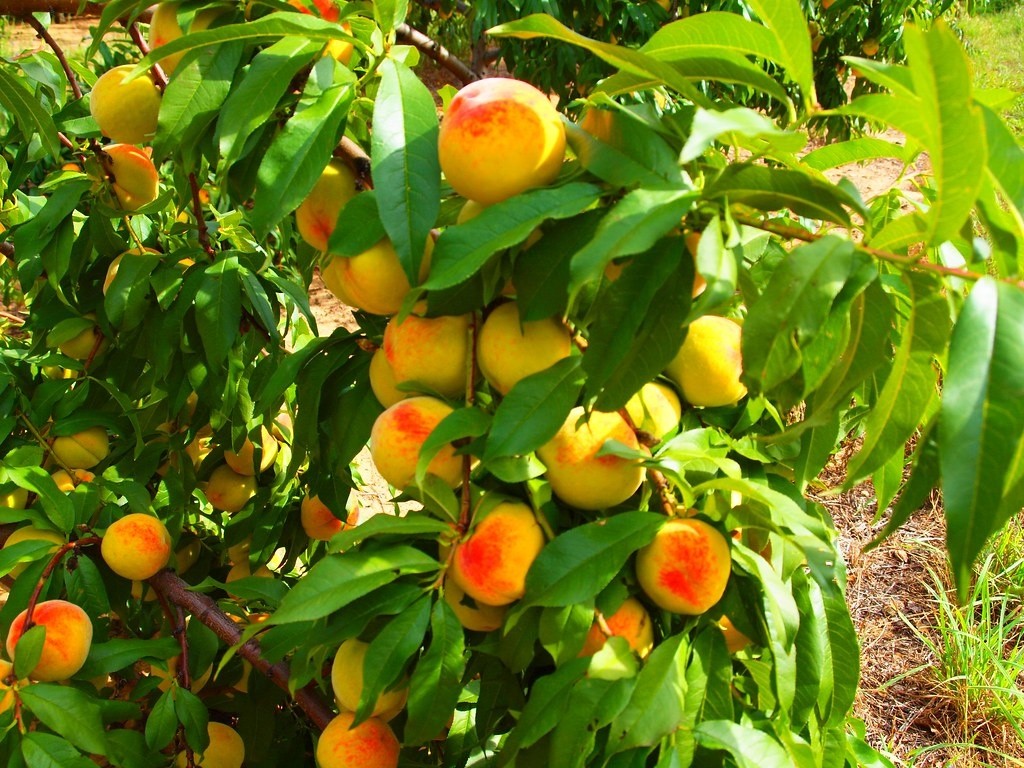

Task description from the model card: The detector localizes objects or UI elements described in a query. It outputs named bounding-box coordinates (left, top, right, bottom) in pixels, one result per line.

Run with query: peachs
left=806, top=0, right=881, bottom=79
left=0, top=1, right=754, bottom=768
